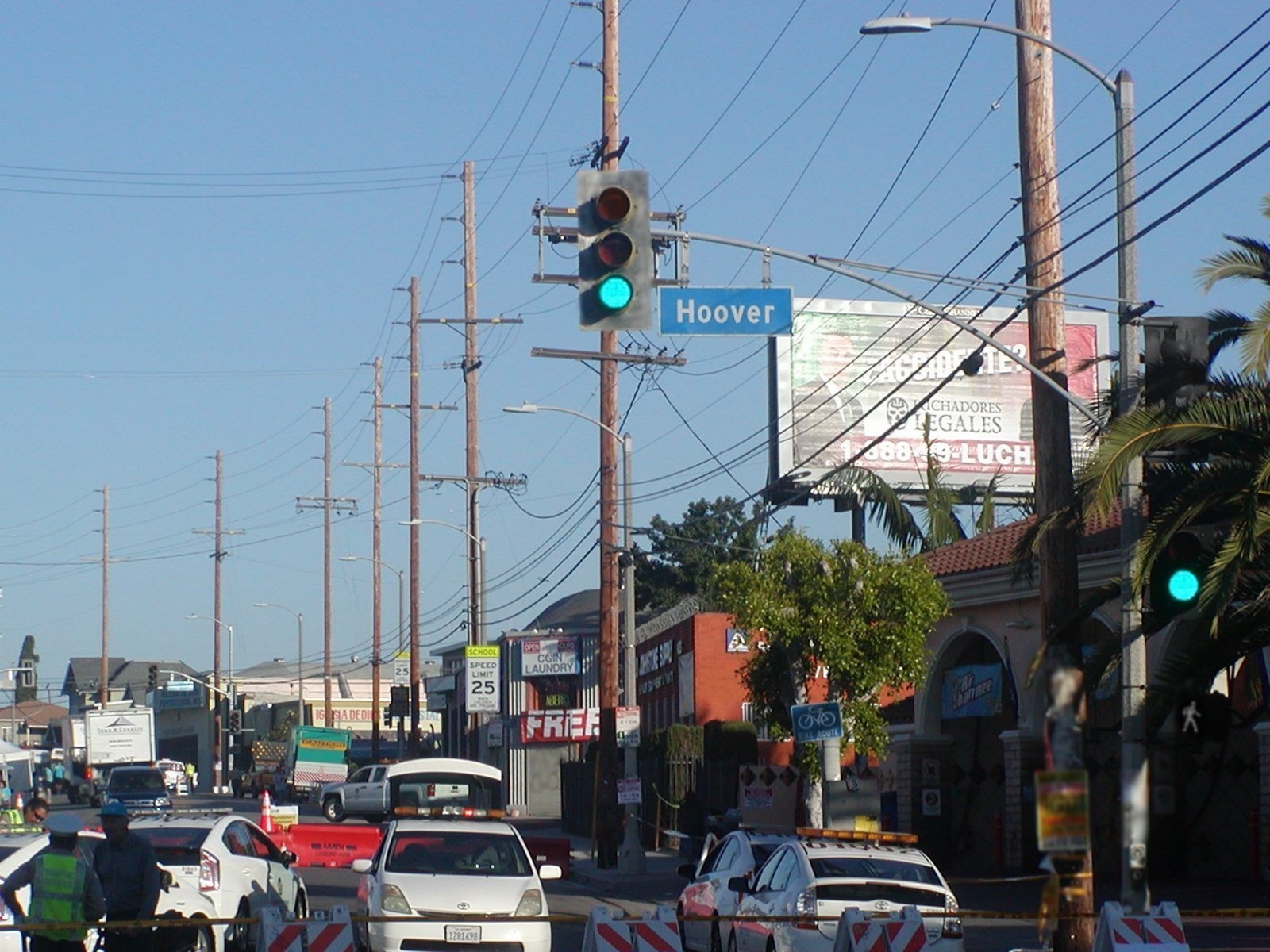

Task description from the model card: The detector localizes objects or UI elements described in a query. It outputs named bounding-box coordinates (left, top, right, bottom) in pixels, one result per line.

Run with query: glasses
left=33, top=809, right=44, bottom=821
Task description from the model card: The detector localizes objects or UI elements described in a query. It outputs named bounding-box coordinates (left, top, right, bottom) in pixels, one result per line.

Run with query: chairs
left=399, top=843, right=432, bottom=869
left=470, top=845, right=500, bottom=869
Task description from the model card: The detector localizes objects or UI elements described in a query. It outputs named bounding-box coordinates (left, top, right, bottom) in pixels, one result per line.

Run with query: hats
left=46, top=814, right=84, bottom=834
left=95, top=802, right=131, bottom=817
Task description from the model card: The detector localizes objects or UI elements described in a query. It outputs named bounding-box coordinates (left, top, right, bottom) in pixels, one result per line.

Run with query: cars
left=96, top=765, right=173, bottom=810
left=673, top=826, right=966, bottom=952
left=156, top=758, right=189, bottom=785
left=0, top=831, right=226, bottom=952
left=347, top=806, right=563, bottom=952
left=125, top=807, right=313, bottom=952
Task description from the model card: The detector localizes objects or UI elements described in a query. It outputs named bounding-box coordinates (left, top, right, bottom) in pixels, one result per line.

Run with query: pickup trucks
left=242, top=763, right=276, bottom=797
left=318, top=763, right=472, bottom=824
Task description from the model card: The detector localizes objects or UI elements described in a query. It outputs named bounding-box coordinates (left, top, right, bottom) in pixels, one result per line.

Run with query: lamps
left=1006, top=621, right=1026, bottom=630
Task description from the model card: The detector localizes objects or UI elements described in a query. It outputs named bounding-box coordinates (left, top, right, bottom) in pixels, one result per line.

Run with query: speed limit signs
left=465, top=645, right=500, bottom=713
left=393, top=653, right=410, bottom=684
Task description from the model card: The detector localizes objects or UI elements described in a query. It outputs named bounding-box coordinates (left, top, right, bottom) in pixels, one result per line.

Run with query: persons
left=230, top=765, right=241, bottom=796
left=0, top=814, right=106, bottom=952
left=0, top=798, right=50, bottom=835
left=457, top=836, right=499, bottom=870
left=28, top=763, right=54, bottom=803
left=53, top=760, right=65, bottom=794
left=184, top=762, right=195, bottom=793
left=1, top=780, right=12, bottom=808
left=91, top=801, right=160, bottom=952
left=273, top=766, right=286, bottom=806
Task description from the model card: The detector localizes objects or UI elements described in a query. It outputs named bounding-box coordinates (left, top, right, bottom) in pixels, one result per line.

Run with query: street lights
left=859, top=14, right=1156, bottom=915
left=505, top=401, right=653, bottom=876
left=184, top=614, right=236, bottom=795
left=398, top=516, right=499, bottom=810
left=253, top=602, right=307, bottom=726
left=339, top=556, right=407, bottom=759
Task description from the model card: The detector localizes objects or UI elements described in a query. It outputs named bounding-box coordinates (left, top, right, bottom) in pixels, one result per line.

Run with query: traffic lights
left=230, top=709, right=243, bottom=736
left=383, top=703, right=393, bottom=729
left=1157, top=531, right=1206, bottom=615
left=581, top=171, right=654, bottom=331
left=148, top=665, right=158, bottom=687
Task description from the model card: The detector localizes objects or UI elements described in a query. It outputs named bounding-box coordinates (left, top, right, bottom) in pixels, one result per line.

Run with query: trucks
left=61, top=708, right=158, bottom=809
left=284, top=725, right=352, bottom=801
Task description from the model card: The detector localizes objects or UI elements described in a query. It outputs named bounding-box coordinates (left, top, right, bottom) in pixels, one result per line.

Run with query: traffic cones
left=259, top=790, right=282, bottom=834
